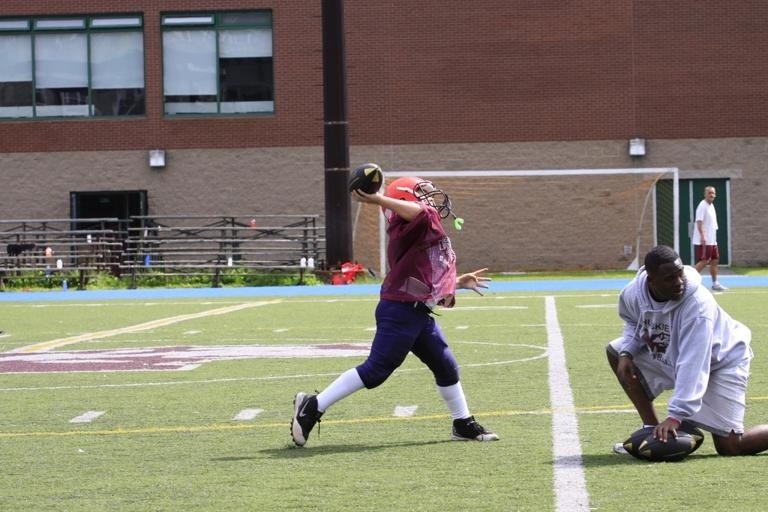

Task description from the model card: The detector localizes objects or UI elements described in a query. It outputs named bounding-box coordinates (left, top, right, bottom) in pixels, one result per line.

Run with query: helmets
left=382, top=177, right=451, bottom=222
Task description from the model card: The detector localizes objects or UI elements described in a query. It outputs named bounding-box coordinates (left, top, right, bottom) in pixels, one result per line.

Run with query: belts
left=411, top=300, right=430, bottom=311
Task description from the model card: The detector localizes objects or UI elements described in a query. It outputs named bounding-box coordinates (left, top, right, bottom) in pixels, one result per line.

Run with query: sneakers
left=712, top=282, right=727, bottom=290
left=451, top=415, right=499, bottom=441
left=290, top=391, right=326, bottom=447
left=613, top=441, right=627, bottom=453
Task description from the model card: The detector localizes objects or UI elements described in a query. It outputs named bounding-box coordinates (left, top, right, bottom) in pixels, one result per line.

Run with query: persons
left=607, top=245, right=768, bottom=456
left=290, top=177, right=500, bottom=446
left=693, top=186, right=728, bottom=291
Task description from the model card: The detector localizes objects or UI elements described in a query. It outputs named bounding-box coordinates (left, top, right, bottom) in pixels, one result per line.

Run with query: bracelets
left=619, top=352, right=632, bottom=359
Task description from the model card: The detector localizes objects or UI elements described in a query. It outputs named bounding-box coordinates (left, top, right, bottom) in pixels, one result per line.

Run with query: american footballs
left=347, top=163, right=383, bottom=197
left=623, top=427, right=704, bottom=462
